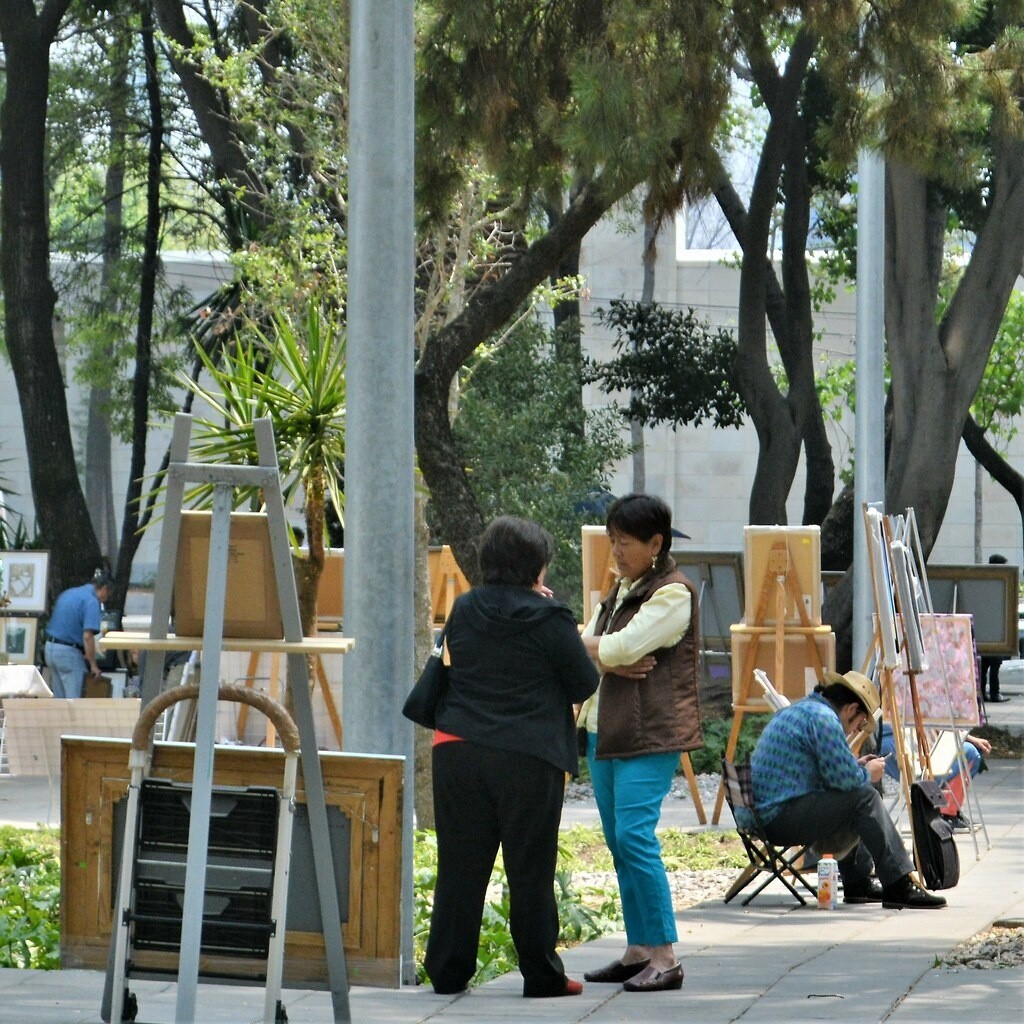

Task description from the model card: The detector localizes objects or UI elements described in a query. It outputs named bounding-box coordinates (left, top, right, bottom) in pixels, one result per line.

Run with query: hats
left=820, top=670, right=878, bottom=731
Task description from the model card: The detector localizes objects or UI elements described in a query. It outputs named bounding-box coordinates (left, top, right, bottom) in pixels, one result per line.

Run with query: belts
left=47, top=637, right=84, bottom=651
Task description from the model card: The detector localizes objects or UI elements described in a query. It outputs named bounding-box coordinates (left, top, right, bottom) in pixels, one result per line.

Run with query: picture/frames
left=0, top=615, right=41, bottom=665
left=1, top=548, right=52, bottom=615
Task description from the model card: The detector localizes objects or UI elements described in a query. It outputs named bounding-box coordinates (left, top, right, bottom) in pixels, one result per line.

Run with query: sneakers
left=957, top=810, right=982, bottom=827
left=941, top=815, right=978, bottom=833
left=882, top=876, right=946, bottom=909
left=844, top=877, right=882, bottom=902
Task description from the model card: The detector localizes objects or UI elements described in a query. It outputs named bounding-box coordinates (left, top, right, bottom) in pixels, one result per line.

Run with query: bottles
left=816, top=854, right=838, bottom=910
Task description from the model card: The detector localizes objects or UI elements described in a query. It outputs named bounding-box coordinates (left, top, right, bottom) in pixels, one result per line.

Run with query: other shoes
left=984, top=694, right=991, bottom=701
left=991, top=690, right=1011, bottom=702
left=567, top=981, right=583, bottom=996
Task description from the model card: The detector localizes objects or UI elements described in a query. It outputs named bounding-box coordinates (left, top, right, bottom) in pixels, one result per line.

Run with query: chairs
left=721, top=749, right=827, bottom=909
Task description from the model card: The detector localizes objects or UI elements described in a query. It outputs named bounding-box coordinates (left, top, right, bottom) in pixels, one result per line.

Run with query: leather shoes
left=624, top=963, right=683, bottom=991
left=584, top=958, right=651, bottom=982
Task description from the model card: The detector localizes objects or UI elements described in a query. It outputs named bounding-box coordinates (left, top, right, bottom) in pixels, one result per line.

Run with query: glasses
left=856, top=713, right=868, bottom=732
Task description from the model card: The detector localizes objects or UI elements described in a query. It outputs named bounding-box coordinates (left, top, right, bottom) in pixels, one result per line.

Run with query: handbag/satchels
left=402, top=630, right=450, bottom=730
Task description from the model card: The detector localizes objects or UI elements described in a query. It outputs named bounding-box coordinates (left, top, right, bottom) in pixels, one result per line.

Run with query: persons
left=749, top=667, right=948, bottom=909
left=424, top=515, right=598, bottom=998
left=881, top=722, right=992, bottom=833
left=578, top=494, right=706, bottom=993
left=979, top=555, right=1011, bottom=703
left=44, top=572, right=115, bottom=699
